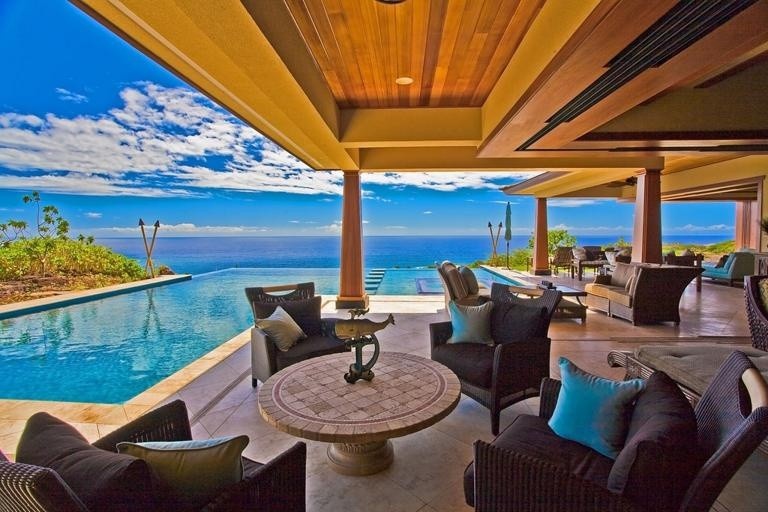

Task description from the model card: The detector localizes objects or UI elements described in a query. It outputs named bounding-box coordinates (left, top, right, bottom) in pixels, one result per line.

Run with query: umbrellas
left=503, top=201, right=512, bottom=268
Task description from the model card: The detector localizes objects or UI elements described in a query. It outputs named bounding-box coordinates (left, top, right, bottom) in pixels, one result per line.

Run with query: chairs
left=702, top=249, right=755, bottom=293
left=433, top=260, right=491, bottom=316
left=551, top=244, right=601, bottom=277
left=1, top=398, right=311, bottom=512
left=245, top=282, right=352, bottom=388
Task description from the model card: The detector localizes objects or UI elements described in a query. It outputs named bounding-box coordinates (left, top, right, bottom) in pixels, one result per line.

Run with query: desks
left=257, top=350, right=463, bottom=477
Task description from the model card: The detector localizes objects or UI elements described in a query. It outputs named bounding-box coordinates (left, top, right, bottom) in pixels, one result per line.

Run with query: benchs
left=569, top=245, right=706, bottom=326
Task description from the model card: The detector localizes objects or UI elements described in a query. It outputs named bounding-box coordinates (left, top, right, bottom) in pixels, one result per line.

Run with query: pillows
left=14, top=411, right=252, bottom=512
left=250, top=295, right=323, bottom=352
left=441, top=261, right=479, bottom=300
